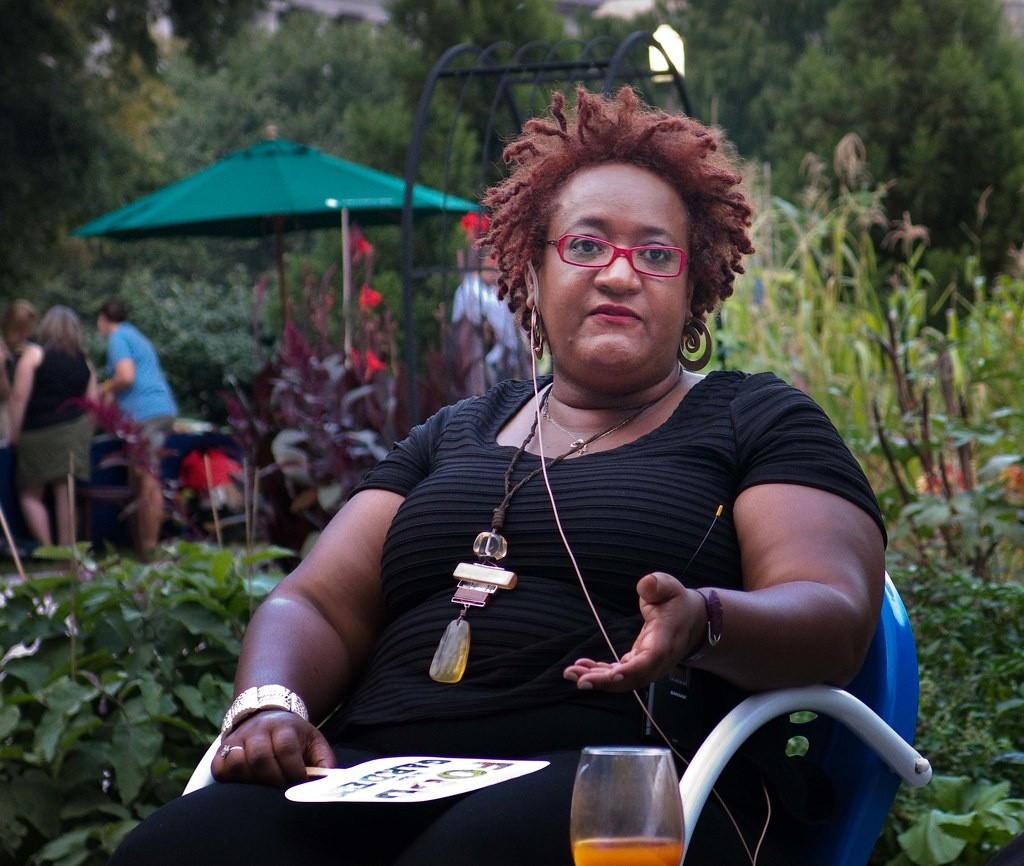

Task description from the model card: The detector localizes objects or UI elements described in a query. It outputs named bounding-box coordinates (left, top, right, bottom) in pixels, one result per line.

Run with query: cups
left=570, top=747, right=685, bottom=866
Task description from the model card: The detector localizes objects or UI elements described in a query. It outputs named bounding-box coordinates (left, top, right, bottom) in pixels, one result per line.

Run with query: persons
left=112, top=83, right=888, bottom=865
left=0, top=299, right=177, bottom=569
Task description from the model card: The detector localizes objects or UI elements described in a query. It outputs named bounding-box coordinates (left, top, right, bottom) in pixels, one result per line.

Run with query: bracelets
left=679, top=590, right=723, bottom=665
left=221, top=684, right=309, bottom=745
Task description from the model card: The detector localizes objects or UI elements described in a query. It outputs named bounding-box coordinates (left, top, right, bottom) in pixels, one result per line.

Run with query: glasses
left=543, top=234, right=691, bottom=278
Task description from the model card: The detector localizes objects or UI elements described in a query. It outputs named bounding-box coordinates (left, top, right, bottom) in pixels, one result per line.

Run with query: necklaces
left=429, top=366, right=683, bottom=684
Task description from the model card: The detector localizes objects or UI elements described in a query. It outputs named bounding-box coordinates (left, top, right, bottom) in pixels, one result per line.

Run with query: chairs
left=182, top=566, right=933, bottom=866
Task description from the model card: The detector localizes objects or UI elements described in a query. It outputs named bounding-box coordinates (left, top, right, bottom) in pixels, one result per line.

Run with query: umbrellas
left=69, top=124, right=487, bottom=345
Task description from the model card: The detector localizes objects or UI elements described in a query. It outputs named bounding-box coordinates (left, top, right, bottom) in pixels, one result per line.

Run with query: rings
left=220, top=746, right=244, bottom=760
